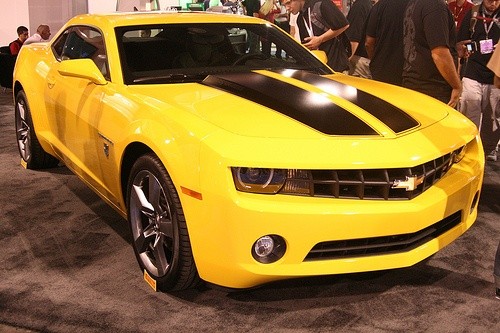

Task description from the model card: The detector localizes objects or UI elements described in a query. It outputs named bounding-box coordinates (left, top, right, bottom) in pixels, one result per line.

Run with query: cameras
left=465, top=41, right=477, bottom=52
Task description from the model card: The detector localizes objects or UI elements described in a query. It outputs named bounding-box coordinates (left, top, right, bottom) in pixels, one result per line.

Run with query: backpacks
left=311, top=0, right=352, bottom=58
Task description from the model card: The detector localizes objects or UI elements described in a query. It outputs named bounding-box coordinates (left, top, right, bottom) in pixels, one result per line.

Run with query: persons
left=191, top=0, right=408, bottom=86
left=402, top=0, right=474, bottom=111
left=9, top=26, right=28, bottom=55
left=493, top=241, right=500, bottom=301
left=446, top=0, right=500, bottom=211
left=23, top=25, right=51, bottom=44
left=141, top=29, right=151, bottom=38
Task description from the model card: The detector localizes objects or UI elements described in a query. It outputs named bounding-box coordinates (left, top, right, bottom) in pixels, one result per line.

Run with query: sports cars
left=13, top=11, right=485, bottom=295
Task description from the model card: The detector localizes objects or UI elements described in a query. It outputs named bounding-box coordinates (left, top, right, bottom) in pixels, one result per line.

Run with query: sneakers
left=485, top=148, right=497, bottom=162
